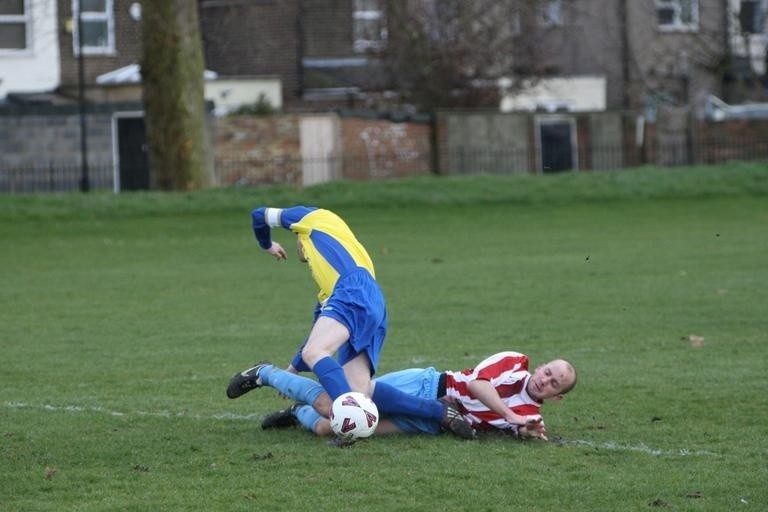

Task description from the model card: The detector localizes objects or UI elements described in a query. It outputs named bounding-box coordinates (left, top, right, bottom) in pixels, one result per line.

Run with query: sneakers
left=438, top=394, right=476, bottom=440
left=227, top=360, right=272, bottom=399
left=261, top=402, right=306, bottom=430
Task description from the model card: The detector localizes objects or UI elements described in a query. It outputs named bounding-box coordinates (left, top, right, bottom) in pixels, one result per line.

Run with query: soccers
left=328, top=392, right=379, bottom=439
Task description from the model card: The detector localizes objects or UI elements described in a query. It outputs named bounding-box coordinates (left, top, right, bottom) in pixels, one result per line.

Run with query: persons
left=224, top=351, right=578, bottom=444
left=250, top=205, right=388, bottom=450
